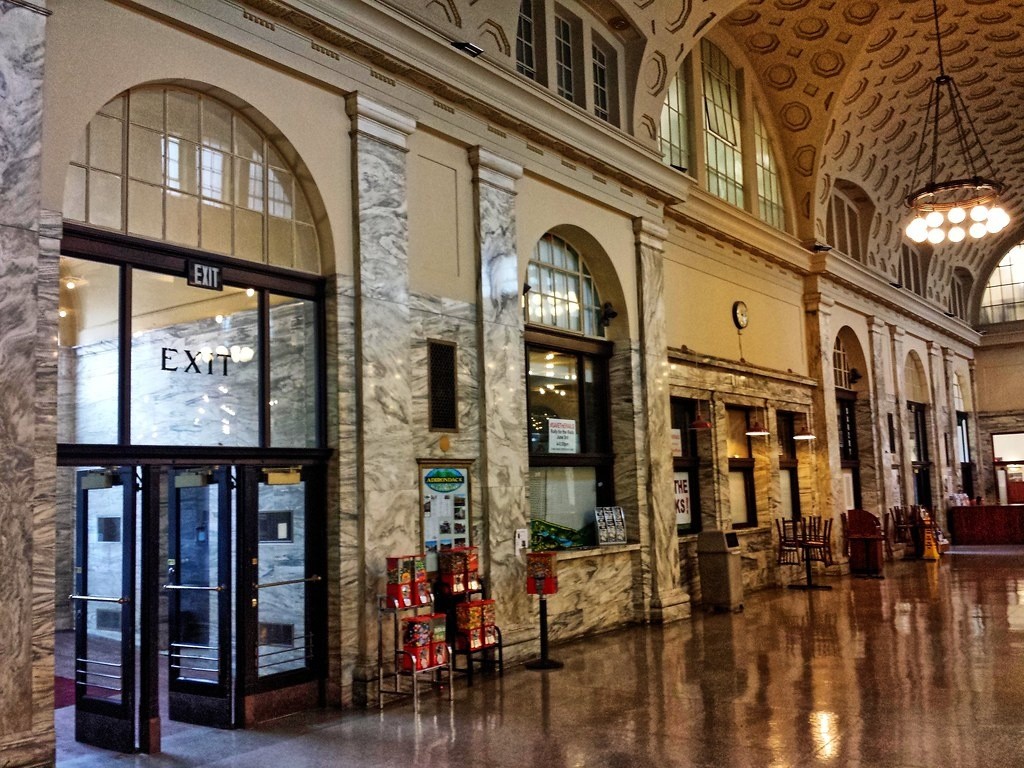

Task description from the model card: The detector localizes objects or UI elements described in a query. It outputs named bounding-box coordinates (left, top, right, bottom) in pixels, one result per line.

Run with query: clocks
left=732, top=301, right=749, bottom=329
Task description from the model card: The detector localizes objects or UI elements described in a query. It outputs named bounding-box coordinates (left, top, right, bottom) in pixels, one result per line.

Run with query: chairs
left=890, top=504, right=941, bottom=543
left=840, top=511, right=894, bottom=558
left=775, top=516, right=834, bottom=568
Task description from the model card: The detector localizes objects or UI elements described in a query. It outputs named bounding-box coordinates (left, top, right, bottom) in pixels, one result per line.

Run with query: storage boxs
left=384, top=546, right=496, bottom=670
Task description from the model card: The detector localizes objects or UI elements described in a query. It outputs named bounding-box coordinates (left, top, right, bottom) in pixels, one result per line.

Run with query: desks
left=851, top=536, right=885, bottom=578
left=900, top=525, right=936, bottom=562
left=785, top=544, right=832, bottom=590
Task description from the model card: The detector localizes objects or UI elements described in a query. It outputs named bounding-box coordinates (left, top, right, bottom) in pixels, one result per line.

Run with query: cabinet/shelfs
left=377, top=578, right=503, bottom=713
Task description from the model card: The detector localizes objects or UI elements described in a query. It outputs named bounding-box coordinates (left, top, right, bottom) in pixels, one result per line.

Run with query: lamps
left=688, top=416, right=713, bottom=430
left=745, top=422, right=770, bottom=436
left=793, top=427, right=816, bottom=441
left=904, top=74, right=1009, bottom=244
left=850, top=368, right=862, bottom=385
